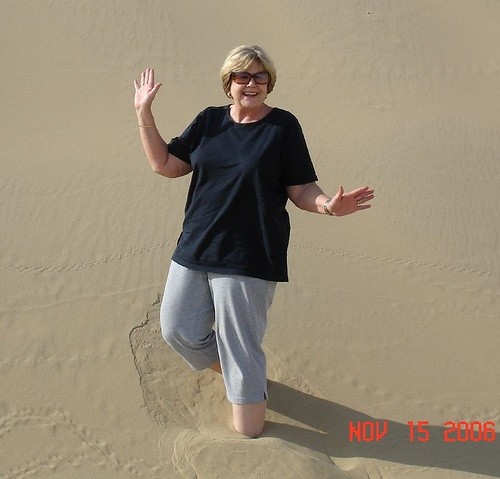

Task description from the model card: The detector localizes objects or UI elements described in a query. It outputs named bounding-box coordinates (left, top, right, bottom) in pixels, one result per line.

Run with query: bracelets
left=138, top=124, right=155, bottom=128
left=323, top=198, right=335, bottom=217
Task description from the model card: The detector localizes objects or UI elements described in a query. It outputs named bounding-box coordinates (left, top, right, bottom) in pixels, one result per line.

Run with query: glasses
left=230, top=71, right=271, bottom=85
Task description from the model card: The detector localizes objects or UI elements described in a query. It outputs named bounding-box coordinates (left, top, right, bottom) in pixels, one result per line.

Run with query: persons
left=132, top=44, right=374, bottom=440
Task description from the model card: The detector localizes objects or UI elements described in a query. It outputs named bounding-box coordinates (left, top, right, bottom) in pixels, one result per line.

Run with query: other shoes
left=233, top=398, right=267, bottom=437
